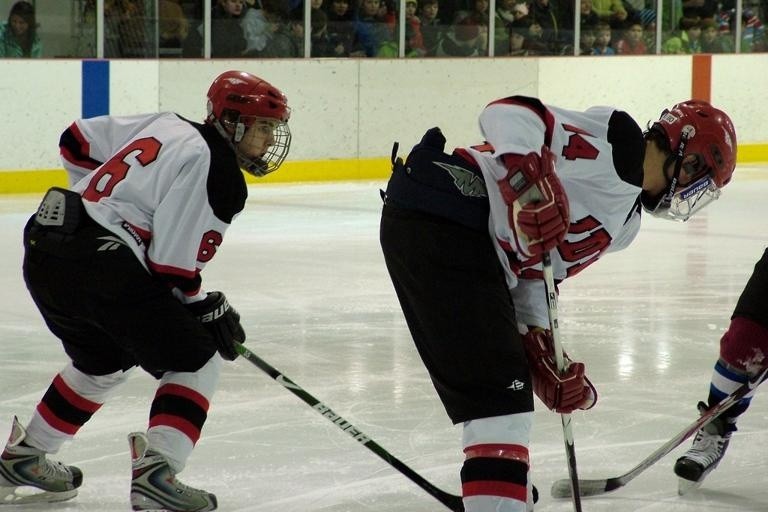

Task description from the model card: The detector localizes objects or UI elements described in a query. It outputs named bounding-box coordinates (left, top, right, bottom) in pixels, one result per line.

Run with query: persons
left=381, top=93, right=740, bottom=512
left=2, top=71, right=293, bottom=511
left=104, top=0, right=768, bottom=57
left=674, top=242, right=768, bottom=482
left=0, top=1, right=42, bottom=59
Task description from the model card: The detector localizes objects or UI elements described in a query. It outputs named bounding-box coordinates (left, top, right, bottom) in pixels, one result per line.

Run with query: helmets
left=652, top=98, right=736, bottom=224
left=203, top=70, right=292, bottom=177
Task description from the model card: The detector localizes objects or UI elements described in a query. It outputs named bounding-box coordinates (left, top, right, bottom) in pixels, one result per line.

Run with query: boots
left=0, top=415, right=82, bottom=492
left=127, top=432, right=216, bottom=512
left=674, top=401, right=736, bottom=483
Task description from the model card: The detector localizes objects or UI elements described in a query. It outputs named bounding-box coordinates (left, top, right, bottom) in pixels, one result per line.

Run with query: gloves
left=495, top=144, right=570, bottom=255
left=522, top=328, right=596, bottom=413
left=184, top=290, right=245, bottom=360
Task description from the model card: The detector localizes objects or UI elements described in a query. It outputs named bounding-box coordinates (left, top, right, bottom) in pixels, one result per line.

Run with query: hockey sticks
left=235, top=341, right=539, bottom=511
left=550, top=362, right=768, bottom=499
